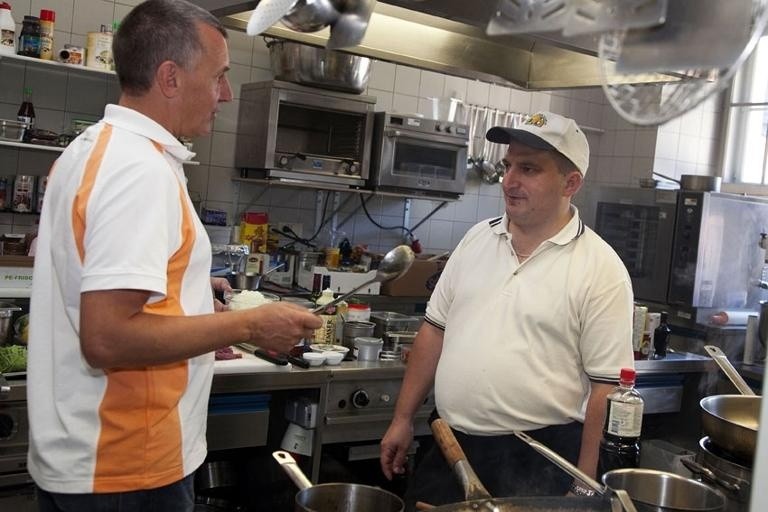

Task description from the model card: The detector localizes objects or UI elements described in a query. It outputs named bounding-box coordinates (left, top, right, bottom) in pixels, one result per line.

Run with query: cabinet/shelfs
left=0, top=52, right=203, bottom=268
left=230, top=177, right=462, bottom=249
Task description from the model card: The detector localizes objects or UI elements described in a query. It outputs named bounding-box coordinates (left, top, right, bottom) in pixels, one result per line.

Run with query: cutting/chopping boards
left=213, top=346, right=292, bottom=374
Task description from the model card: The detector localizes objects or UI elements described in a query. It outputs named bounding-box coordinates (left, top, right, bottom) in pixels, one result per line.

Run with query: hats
left=486, top=112, right=591, bottom=178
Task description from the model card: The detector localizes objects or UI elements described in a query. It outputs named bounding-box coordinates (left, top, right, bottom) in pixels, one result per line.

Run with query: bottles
left=86, top=31, right=113, bottom=72
left=0, top=173, right=6, bottom=212
left=600, top=369, right=644, bottom=469
left=18, top=15, right=39, bottom=55
left=12, top=175, right=37, bottom=214
left=40, top=10, right=55, bottom=59
left=654, top=312, right=671, bottom=356
left=316, top=290, right=336, bottom=331
left=16, top=87, right=35, bottom=142
left=334, top=296, right=348, bottom=319
left=640, top=332, right=652, bottom=358
left=0, top=3, right=15, bottom=57
left=38, top=177, right=47, bottom=214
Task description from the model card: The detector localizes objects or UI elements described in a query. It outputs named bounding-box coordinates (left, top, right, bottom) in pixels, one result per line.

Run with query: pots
left=272, top=449, right=406, bottom=509
left=698, top=345, right=764, bottom=460
left=422, top=418, right=627, bottom=509
left=510, top=430, right=729, bottom=508
left=228, top=263, right=287, bottom=291
left=653, top=169, right=722, bottom=193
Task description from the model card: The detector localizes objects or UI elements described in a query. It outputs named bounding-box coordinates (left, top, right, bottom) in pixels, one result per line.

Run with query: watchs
left=569, top=483, right=596, bottom=497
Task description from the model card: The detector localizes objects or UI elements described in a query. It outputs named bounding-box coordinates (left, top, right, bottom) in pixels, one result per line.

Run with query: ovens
left=577, top=184, right=763, bottom=324
left=234, top=80, right=379, bottom=188
left=368, top=110, right=471, bottom=197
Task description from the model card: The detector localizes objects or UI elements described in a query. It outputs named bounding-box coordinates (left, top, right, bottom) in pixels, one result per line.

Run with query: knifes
left=233, top=342, right=288, bottom=365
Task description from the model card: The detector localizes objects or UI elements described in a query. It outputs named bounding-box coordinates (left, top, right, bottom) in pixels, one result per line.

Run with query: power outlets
left=278, top=222, right=304, bottom=240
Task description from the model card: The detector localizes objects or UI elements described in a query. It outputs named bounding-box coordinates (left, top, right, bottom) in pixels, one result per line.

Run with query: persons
left=380, top=110, right=634, bottom=512
left=25, top=0, right=323, bottom=512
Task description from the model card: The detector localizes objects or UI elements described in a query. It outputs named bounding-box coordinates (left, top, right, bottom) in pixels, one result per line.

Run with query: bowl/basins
left=322, top=350, right=345, bottom=366
left=356, top=336, right=383, bottom=362
left=340, top=321, right=375, bottom=358
left=370, top=309, right=420, bottom=343
left=308, top=342, right=350, bottom=361
left=303, top=351, right=327, bottom=367
left=639, top=177, right=658, bottom=188
left=264, top=34, right=375, bottom=96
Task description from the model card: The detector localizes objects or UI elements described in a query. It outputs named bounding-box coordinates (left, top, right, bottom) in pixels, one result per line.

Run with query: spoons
left=462, top=105, right=530, bottom=185
left=311, top=243, right=415, bottom=316
